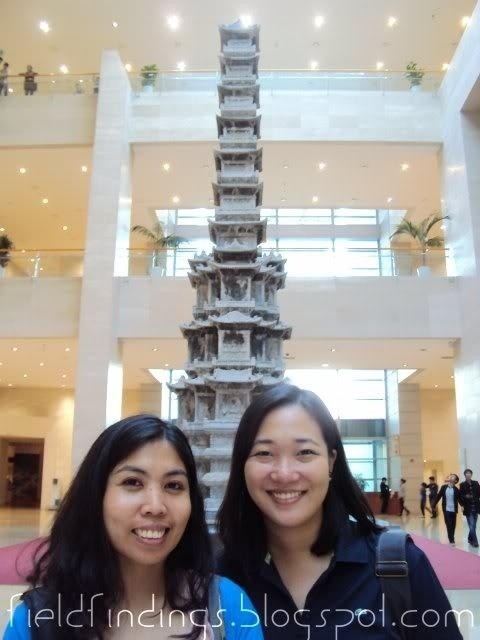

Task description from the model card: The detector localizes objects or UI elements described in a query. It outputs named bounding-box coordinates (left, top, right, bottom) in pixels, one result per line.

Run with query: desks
left=365, top=490, right=401, bottom=516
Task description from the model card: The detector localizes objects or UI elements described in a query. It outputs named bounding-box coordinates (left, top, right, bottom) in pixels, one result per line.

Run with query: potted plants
left=388, top=210, right=452, bottom=277
left=139, top=63, right=159, bottom=92
left=354, top=474, right=368, bottom=492
left=404, top=61, right=426, bottom=91
left=130, top=221, right=189, bottom=277
left=0, top=234, right=14, bottom=277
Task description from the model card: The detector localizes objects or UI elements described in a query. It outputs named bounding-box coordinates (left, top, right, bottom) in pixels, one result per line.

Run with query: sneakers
left=398, top=510, right=437, bottom=518
left=449, top=536, right=479, bottom=547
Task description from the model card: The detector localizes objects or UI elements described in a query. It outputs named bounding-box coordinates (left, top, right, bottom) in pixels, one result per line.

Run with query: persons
left=459, top=469, right=480, bottom=547
left=426, top=476, right=438, bottom=518
left=397, top=478, right=410, bottom=516
left=207, top=385, right=464, bottom=638
left=418, top=481, right=431, bottom=518
left=432, top=473, right=460, bottom=545
left=380, top=477, right=391, bottom=515
left=4, top=412, right=266, bottom=639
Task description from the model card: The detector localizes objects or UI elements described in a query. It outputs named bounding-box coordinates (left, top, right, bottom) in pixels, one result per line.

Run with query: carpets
left=0, top=533, right=480, bottom=590
